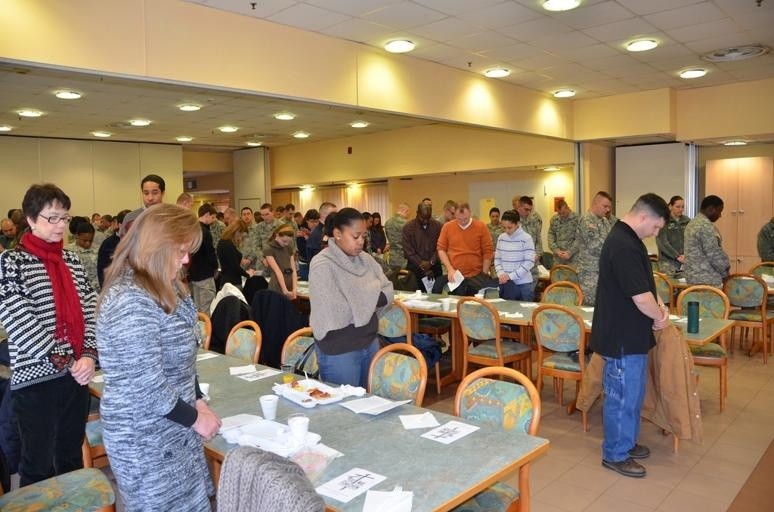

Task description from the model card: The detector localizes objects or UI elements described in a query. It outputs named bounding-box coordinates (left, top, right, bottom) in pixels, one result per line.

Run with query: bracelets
left=659, top=303, right=669, bottom=312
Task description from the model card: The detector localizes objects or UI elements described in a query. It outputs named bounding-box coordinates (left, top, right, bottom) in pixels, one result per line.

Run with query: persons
left=682, top=195, right=731, bottom=291
left=307, top=207, right=394, bottom=385
left=1, top=208, right=31, bottom=252
left=65, top=173, right=165, bottom=289
left=588, top=193, right=671, bottom=477
left=0, top=181, right=100, bottom=489
left=757, top=216, right=774, bottom=267
left=656, top=196, right=692, bottom=278
left=548, top=190, right=617, bottom=306
left=363, top=196, right=543, bottom=349
left=175, top=193, right=337, bottom=316
left=95, top=203, right=223, bottom=512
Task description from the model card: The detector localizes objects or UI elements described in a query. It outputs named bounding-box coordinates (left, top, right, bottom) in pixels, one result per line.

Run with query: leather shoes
left=599, top=457, right=648, bottom=478
left=628, top=443, right=650, bottom=459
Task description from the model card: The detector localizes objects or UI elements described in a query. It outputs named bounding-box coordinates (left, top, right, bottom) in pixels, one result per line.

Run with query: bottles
left=684, top=300, right=701, bottom=333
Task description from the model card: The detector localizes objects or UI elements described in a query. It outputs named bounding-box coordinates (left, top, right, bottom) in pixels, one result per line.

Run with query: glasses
left=37, top=212, right=72, bottom=225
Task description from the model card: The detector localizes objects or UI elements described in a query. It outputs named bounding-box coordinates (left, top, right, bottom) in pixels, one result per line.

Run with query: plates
left=414, top=301, right=437, bottom=309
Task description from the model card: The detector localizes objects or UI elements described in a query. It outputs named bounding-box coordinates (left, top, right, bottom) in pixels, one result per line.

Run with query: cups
left=441, top=297, right=451, bottom=312
left=258, top=393, right=280, bottom=421
left=285, top=412, right=311, bottom=448
left=196, top=383, right=212, bottom=403
left=281, top=364, right=295, bottom=383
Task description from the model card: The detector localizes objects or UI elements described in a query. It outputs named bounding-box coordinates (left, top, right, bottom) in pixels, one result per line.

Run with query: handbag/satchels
left=377, top=324, right=443, bottom=371
